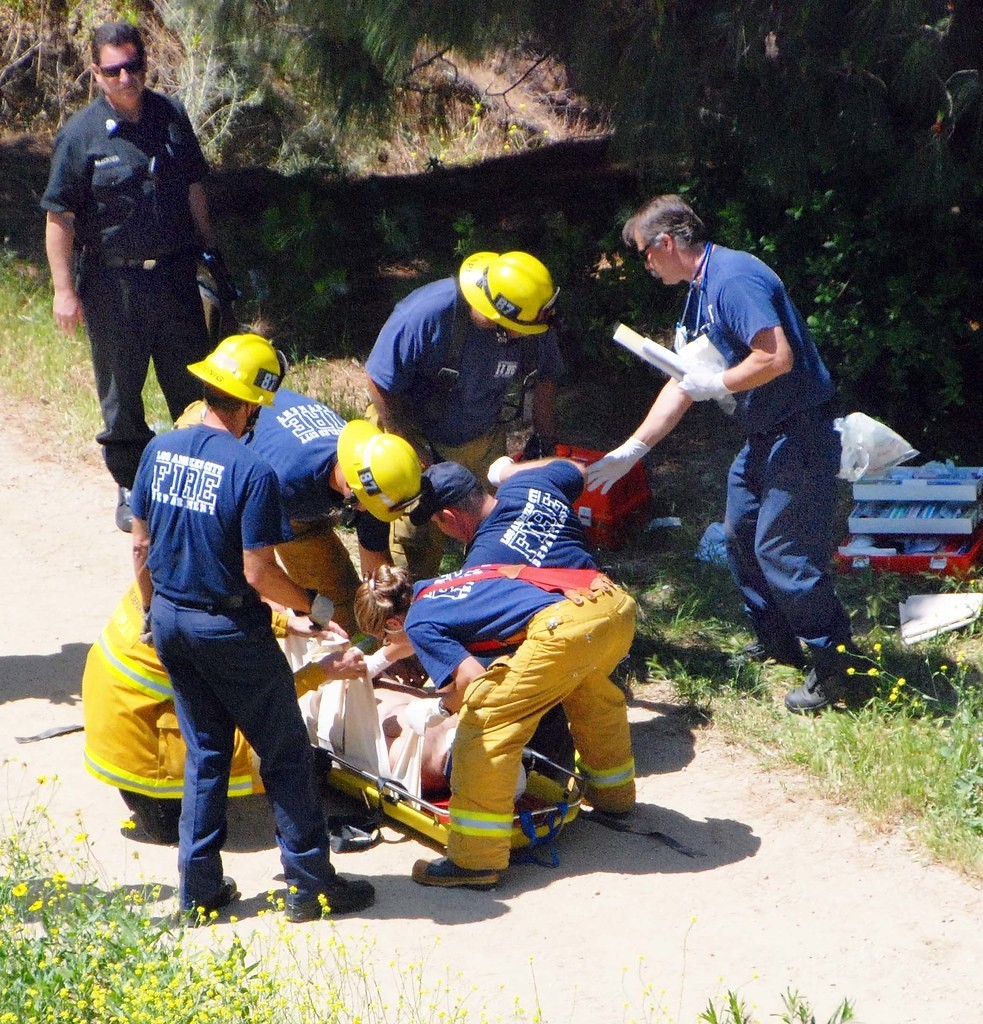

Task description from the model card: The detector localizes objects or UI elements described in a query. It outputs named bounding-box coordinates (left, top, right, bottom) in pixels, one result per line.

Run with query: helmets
left=460, top=251, right=562, bottom=334
left=337, top=420, right=422, bottom=522
left=186, top=334, right=288, bottom=406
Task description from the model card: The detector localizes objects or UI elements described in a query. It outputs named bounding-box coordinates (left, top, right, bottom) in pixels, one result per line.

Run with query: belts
left=103, top=253, right=178, bottom=270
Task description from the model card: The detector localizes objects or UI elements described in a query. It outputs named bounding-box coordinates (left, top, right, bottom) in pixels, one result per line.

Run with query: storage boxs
left=513, top=443, right=649, bottom=524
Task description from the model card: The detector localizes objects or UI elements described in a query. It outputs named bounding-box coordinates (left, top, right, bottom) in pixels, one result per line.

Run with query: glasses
left=636, top=237, right=657, bottom=262
left=97, top=57, right=144, bottom=77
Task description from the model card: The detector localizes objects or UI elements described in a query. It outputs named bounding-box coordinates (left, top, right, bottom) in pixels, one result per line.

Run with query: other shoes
left=784, top=647, right=874, bottom=715
left=118, top=788, right=179, bottom=845
left=115, top=484, right=133, bottom=532
left=411, top=856, right=499, bottom=891
left=286, top=875, right=375, bottom=923
left=742, top=641, right=768, bottom=662
left=181, top=875, right=237, bottom=928
left=568, top=773, right=629, bottom=819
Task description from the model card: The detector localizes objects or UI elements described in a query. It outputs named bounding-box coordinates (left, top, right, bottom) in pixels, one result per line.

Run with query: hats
left=409, top=461, right=477, bottom=525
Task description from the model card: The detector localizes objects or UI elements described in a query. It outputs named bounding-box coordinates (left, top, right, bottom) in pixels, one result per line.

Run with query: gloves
left=363, top=646, right=393, bottom=680
left=677, top=362, right=737, bottom=402
left=399, top=698, right=447, bottom=736
left=585, top=436, right=651, bottom=496
left=487, top=455, right=514, bottom=487
left=293, top=588, right=335, bottom=633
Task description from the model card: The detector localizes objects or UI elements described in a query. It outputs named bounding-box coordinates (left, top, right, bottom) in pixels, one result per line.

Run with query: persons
left=41, top=23, right=227, bottom=531
left=586, top=194, right=871, bottom=712
left=81, top=251, right=637, bottom=921
